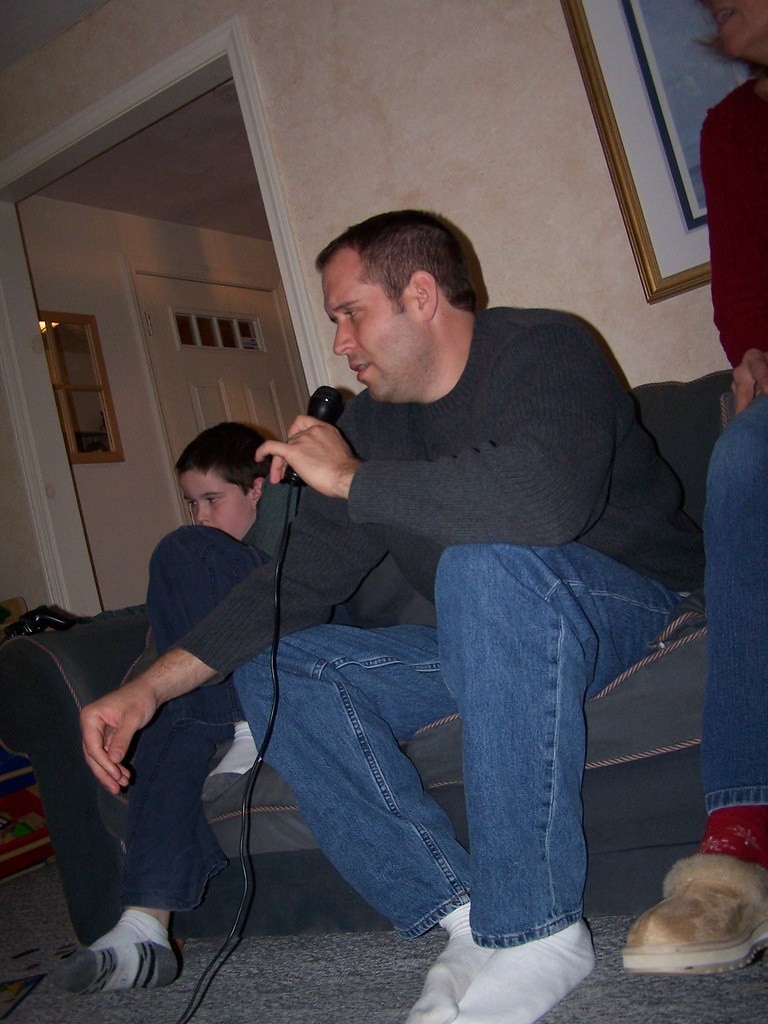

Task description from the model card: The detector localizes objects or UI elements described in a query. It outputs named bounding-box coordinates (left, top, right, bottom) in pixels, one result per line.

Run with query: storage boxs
left=0, top=598, right=55, bottom=883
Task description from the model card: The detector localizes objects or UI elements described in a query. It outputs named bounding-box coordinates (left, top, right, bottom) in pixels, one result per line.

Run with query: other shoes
left=620, top=853, right=768, bottom=977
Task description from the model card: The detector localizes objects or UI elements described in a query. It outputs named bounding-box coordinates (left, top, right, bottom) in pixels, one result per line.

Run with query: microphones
left=284, top=385, right=344, bottom=487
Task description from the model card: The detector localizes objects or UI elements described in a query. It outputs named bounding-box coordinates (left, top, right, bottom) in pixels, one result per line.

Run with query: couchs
left=0, top=367, right=743, bottom=951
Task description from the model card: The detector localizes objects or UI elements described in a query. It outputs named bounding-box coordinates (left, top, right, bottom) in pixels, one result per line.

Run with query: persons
left=621, top=0, right=768, bottom=976
left=78, top=211, right=703, bottom=1024
left=55, top=423, right=350, bottom=994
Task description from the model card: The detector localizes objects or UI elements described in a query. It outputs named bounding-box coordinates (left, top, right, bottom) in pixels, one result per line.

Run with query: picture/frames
left=560, top=0, right=758, bottom=303
